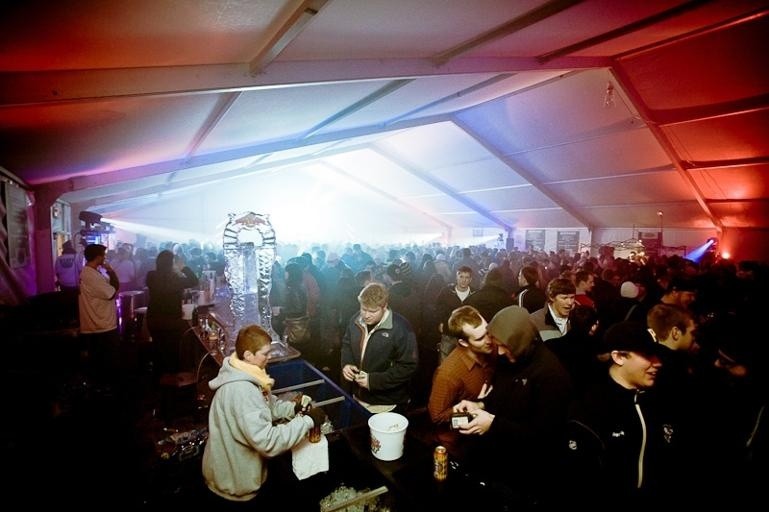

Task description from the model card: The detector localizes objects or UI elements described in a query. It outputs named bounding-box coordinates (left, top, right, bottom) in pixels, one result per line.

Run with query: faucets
left=208, top=312, right=230, bottom=356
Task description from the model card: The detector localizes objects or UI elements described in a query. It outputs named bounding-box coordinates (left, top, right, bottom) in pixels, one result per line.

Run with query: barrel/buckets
left=369, top=411, right=409, bottom=461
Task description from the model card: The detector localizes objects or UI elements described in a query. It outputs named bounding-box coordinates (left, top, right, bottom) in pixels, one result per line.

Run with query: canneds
left=433, top=445, right=449, bottom=482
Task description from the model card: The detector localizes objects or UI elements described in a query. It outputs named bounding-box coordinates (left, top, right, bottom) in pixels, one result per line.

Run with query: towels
left=291, top=431, right=331, bottom=481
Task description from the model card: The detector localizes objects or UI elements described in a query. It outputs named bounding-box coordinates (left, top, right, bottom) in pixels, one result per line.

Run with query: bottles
left=184, top=290, right=192, bottom=304
left=199, top=317, right=209, bottom=339
left=309, top=424, right=321, bottom=442
left=208, top=321, right=218, bottom=356
left=191, top=304, right=199, bottom=326
left=159, top=428, right=208, bottom=461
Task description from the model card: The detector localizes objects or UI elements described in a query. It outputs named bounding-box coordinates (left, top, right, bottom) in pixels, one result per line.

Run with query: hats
left=668, top=273, right=694, bottom=292
left=608, top=322, right=670, bottom=357
left=621, top=281, right=639, bottom=297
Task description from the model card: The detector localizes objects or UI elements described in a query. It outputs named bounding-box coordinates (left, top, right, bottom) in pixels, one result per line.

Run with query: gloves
left=294, top=401, right=311, bottom=415
left=308, top=407, right=325, bottom=426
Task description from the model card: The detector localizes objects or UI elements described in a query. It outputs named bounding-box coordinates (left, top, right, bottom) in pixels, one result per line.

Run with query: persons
left=268, top=241, right=769, bottom=512
left=202, top=326, right=325, bottom=512
left=55, top=234, right=225, bottom=382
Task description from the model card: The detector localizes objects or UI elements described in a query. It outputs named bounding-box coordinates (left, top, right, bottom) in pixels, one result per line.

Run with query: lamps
left=701, top=236, right=719, bottom=253
left=601, top=79, right=617, bottom=110
